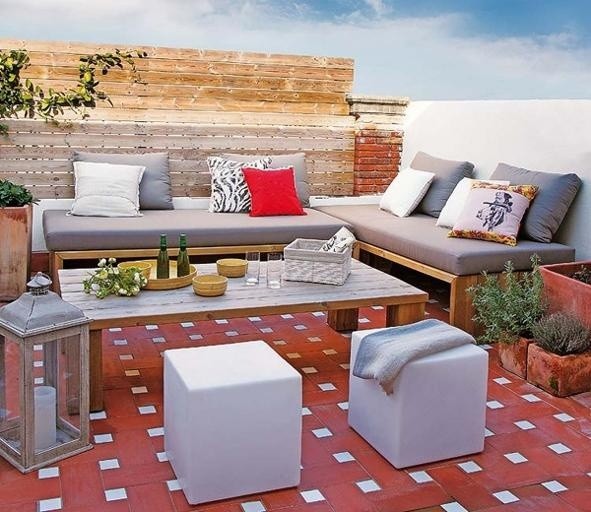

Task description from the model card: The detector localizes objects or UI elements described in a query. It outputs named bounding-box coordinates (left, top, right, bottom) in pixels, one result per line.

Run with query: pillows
left=71, top=162, right=146, bottom=216
left=241, top=166, right=307, bottom=215
left=380, top=167, right=436, bottom=217
left=207, top=156, right=273, bottom=213
left=447, top=181, right=539, bottom=246
left=73, top=152, right=174, bottom=210
left=411, top=151, right=475, bottom=218
left=436, top=177, right=510, bottom=228
left=222, top=152, right=310, bottom=208
left=488, top=163, right=581, bottom=244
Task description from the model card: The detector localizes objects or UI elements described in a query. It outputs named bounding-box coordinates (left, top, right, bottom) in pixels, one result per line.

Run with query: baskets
left=283, top=238, right=352, bottom=286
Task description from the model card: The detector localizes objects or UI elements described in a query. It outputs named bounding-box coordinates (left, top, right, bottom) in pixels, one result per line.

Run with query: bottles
left=177, top=233, right=190, bottom=276
left=157, top=233, right=169, bottom=279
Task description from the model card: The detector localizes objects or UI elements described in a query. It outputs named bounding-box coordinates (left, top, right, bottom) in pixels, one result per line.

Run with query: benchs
left=42, top=209, right=359, bottom=298
left=312, top=204, right=576, bottom=344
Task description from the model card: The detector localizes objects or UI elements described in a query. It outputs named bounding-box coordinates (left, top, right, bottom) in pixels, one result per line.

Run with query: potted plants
left=527, top=313, right=591, bottom=398
left=536, top=261, right=591, bottom=330
left=0, top=178, right=40, bottom=301
left=462, top=251, right=548, bottom=379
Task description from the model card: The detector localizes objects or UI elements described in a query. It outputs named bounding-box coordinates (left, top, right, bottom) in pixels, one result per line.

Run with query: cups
left=266, top=252, right=283, bottom=288
left=246, top=251, right=261, bottom=286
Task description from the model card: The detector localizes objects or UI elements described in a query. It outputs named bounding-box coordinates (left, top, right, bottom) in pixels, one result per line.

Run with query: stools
left=347, top=324, right=489, bottom=470
left=163, top=340, right=302, bottom=506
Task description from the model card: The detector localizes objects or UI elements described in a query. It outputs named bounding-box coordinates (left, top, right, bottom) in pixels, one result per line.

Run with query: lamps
left=0, top=272, right=95, bottom=475
